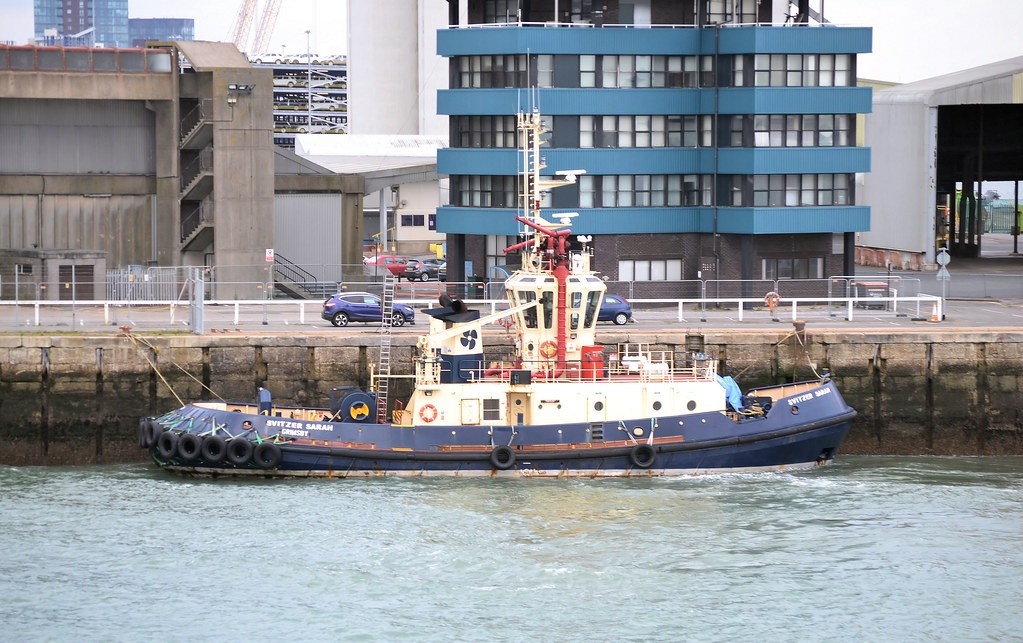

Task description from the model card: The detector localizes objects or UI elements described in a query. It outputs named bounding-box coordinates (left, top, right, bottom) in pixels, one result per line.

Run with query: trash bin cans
left=856, top=281, right=890, bottom=310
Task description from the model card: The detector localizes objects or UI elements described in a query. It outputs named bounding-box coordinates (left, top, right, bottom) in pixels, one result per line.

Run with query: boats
left=137, top=104, right=859, bottom=481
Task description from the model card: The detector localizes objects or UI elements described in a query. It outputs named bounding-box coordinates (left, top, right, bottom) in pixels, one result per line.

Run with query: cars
left=364, top=255, right=447, bottom=283
left=574, top=293, right=632, bottom=325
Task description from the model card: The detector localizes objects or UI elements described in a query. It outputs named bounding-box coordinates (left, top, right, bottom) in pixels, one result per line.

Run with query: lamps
left=228, top=84, right=255, bottom=92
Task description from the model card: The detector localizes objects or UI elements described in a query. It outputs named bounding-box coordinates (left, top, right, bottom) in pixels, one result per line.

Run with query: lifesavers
left=254, top=442, right=281, bottom=470
left=539, top=339, right=557, bottom=358
left=490, top=444, right=516, bottom=469
left=177, top=432, right=201, bottom=462
left=136, top=419, right=160, bottom=449
left=630, top=442, right=657, bottom=468
left=227, top=437, right=252, bottom=466
left=201, top=435, right=225, bottom=464
left=764, top=291, right=780, bottom=306
left=418, top=403, right=438, bottom=423
left=157, top=428, right=179, bottom=458
left=497, top=313, right=514, bottom=327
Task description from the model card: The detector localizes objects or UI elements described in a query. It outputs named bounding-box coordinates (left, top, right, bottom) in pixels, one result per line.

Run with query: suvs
left=320, top=291, right=415, bottom=328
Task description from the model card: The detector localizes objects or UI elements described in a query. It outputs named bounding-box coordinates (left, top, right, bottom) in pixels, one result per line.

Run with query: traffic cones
left=931, top=303, right=938, bottom=321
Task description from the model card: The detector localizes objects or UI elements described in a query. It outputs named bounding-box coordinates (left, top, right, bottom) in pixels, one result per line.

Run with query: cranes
left=229, top=0, right=283, bottom=54
